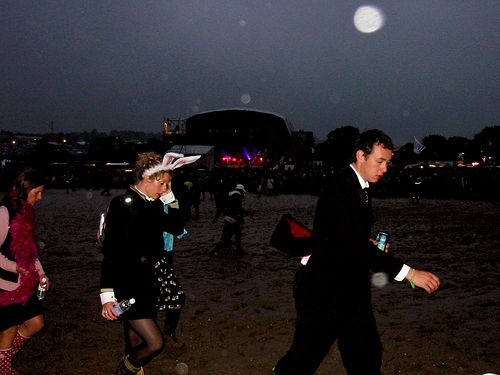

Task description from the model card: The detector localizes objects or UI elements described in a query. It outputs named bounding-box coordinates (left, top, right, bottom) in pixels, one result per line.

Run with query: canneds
left=375, top=232, right=388, bottom=251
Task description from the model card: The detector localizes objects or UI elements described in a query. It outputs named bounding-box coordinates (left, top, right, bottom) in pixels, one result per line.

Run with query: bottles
left=113, top=298, right=136, bottom=315
left=37, top=278, right=47, bottom=301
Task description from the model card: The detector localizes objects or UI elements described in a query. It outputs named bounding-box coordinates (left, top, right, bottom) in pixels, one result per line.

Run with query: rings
left=105, top=311, right=108, bottom=313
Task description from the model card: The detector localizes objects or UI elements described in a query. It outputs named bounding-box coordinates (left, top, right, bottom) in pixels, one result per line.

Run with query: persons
left=271, top=129, right=441, bottom=375
left=0, top=151, right=421, bottom=375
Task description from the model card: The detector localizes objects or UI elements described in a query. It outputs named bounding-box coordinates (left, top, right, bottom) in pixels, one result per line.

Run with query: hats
left=236, top=184, right=246, bottom=193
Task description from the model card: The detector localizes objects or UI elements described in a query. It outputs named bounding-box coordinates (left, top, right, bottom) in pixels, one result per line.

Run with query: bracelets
left=409, top=268, right=415, bottom=288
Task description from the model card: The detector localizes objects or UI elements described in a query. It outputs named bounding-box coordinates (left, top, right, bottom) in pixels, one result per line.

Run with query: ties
left=362, top=187, right=369, bottom=210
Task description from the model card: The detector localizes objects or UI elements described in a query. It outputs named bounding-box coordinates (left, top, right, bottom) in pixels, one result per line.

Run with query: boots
left=0, top=348, right=18, bottom=375
left=11, top=330, right=32, bottom=358
left=116, top=354, right=146, bottom=375
